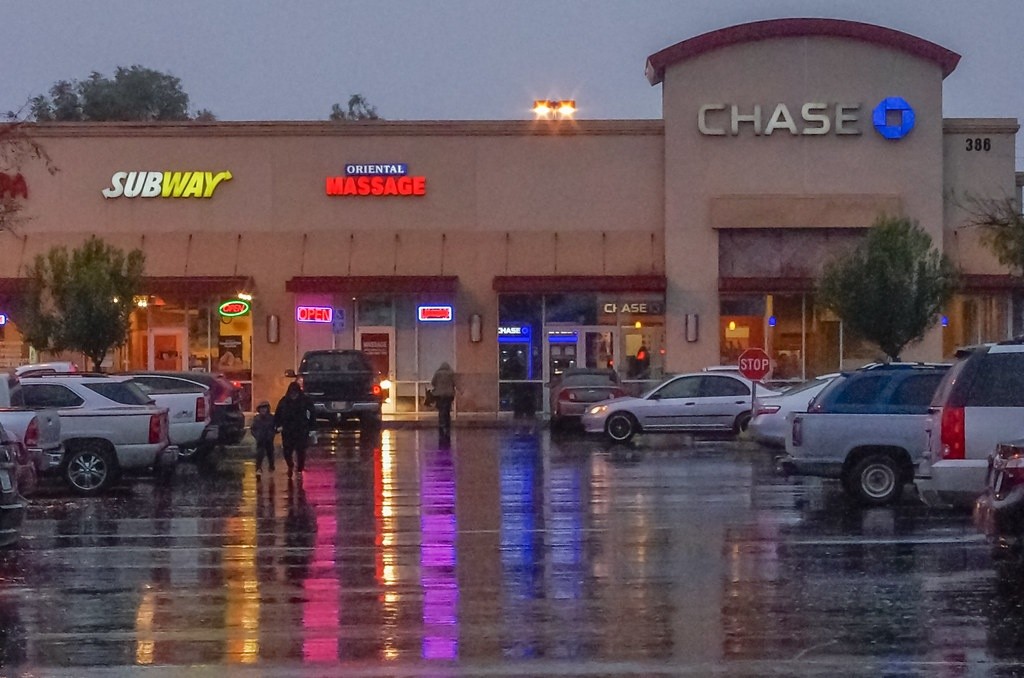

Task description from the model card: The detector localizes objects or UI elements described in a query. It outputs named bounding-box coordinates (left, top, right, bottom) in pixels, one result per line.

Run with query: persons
left=250, top=400, right=281, bottom=473
left=431, top=362, right=464, bottom=429
left=275, top=381, right=317, bottom=476
left=508, top=357, right=538, bottom=436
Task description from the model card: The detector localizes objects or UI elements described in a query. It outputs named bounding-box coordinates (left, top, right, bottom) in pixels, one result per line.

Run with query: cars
left=577, top=372, right=782, bottom=447
left=701, top=365, right=770, bottom=389
left=13, top=360, right=77, bottom=377
left=544, top=369, right=627, bottom=429
left=974, top=435, right=1024, bottom=543
left=747, top=372, right=842, bottom=446
left=216, top=369, right=251, bottom=400
left=0, top=415, right=32, bottom=551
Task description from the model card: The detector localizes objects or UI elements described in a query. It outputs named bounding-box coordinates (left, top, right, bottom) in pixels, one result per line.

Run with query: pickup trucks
left=775, top=412, right=932, bottom=506
left=283, top=349, right=391, bottom=432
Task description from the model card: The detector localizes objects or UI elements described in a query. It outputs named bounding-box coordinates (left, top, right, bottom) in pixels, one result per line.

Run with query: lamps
left=533, top=100, right=576, bottom=115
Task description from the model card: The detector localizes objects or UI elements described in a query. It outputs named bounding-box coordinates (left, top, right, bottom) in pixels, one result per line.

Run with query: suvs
left=0, top=369, right=182, bottom=495
left=805, top=359, right=956, bottom=415
left=912, top=336, right=1024, bottom=500
left=114, top=373, right=247, bottom=454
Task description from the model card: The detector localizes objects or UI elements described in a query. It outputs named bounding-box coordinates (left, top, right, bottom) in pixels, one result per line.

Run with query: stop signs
left=738, top=348, right=772, bottom=382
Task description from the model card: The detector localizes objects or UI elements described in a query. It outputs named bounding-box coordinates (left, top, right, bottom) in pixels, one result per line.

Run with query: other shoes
left=298, top=465, right=302, bottom=475
left=256, top=469, right=262, bottom=478
left=268, top=467, right=275, bottom=472
left=438, top=434, right=451, bottom=445
left=287, top=463, right=294, bottom=477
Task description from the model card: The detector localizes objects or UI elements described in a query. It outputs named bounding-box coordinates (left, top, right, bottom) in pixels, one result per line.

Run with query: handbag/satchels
left=308, top=429, right=318, bottom=445
